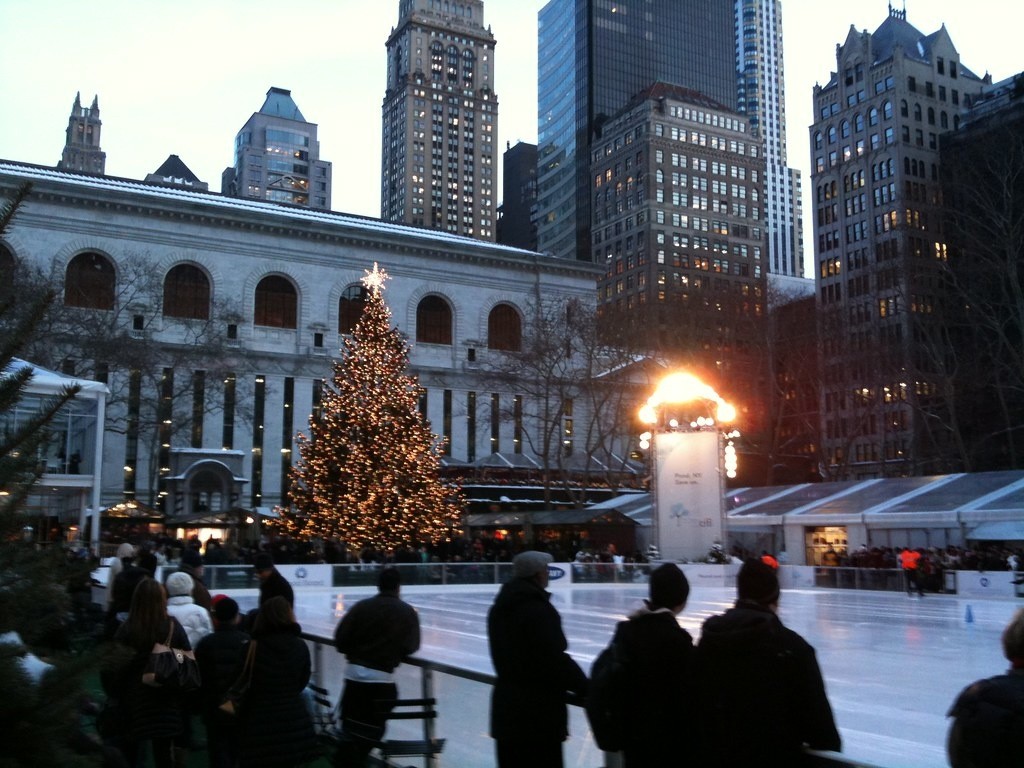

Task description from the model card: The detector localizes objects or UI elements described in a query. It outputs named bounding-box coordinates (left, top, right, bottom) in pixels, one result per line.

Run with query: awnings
left=965, top=521, right=1024, bottom=540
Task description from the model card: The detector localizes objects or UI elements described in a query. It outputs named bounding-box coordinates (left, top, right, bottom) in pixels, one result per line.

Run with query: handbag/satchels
left=141, top=619, right=201, bottom=693
left=217, top=640, right=256, bottom=716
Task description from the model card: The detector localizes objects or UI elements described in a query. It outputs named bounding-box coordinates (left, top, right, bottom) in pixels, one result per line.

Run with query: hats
left=648, top=562, right=690, bottom=610
left=735, top=556, right=781, bottom=605
left=166, top=572, right=194, bottom=595
left=513, top=551, right=553, bottom=572
left=212, top=594, right=240, bottom=623
left=255, top=552, right=274, bottom=569
left=180, top=550, right=204, bottom=568
left=117, top=543, right=133, bottom=558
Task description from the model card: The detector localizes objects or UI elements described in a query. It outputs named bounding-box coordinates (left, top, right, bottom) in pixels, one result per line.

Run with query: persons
left=946, top=607, right=1024, bottom=768
left=69, top=449, right=81, bottom=474
left=0, top=519, right=843, bottom=768
left=823, top=540, right=1024, bottom=598
